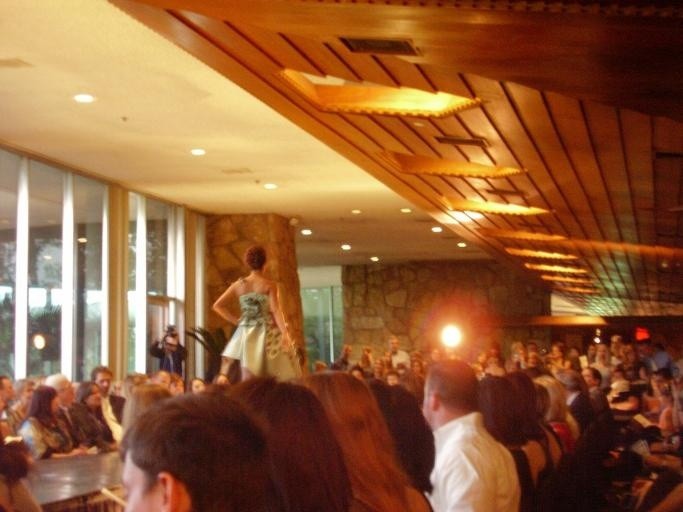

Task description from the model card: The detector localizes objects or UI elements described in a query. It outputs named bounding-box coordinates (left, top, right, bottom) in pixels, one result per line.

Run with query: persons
left=212, top=245, right=300, bottom=384
left=0, top=327, right=683, bottom=512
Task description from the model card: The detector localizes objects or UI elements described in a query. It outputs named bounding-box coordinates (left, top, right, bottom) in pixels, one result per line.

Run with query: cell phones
left=103, top=487, right=126, bottom=507
